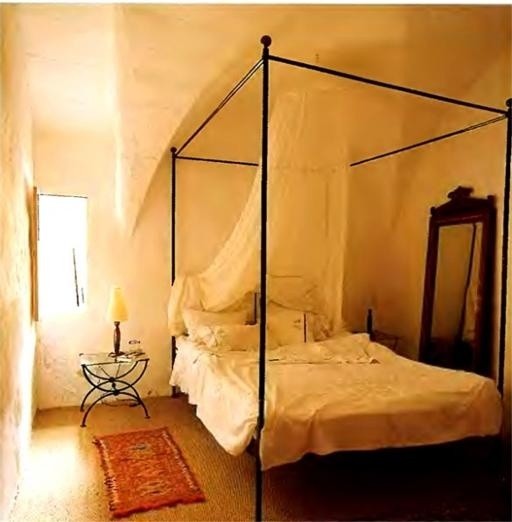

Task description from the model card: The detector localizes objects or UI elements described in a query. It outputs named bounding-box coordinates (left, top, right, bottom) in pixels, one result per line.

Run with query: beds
left=166, top=27, right=512, bottom=522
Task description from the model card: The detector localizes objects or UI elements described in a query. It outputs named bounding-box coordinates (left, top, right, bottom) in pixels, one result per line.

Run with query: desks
left=74, top=353, right=155, bottom=428
left=350, top=330, right=403, bottom=355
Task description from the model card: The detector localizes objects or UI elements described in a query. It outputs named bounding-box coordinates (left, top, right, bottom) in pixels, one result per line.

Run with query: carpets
left=90, top=424, right=207, bottom=518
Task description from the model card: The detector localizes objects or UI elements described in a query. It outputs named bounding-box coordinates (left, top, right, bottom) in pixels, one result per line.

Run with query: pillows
left=270, top=298, right=317, bottom=350
left=177, top=303, right=250, bottom=343
left=193, top=320, right=279, bottom=354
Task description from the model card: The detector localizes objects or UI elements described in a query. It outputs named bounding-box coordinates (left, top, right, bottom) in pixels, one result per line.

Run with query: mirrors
left=416, top=183, right=498, bottom=381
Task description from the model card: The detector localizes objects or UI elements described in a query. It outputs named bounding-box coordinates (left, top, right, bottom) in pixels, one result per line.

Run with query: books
left=116, top=351, right=146, bottom=361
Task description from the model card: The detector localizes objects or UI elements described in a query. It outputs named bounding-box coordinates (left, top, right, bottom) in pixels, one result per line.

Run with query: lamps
left=361, top=285, right=376, bottom=333
left=104, top=284, right=126, bottom=357
